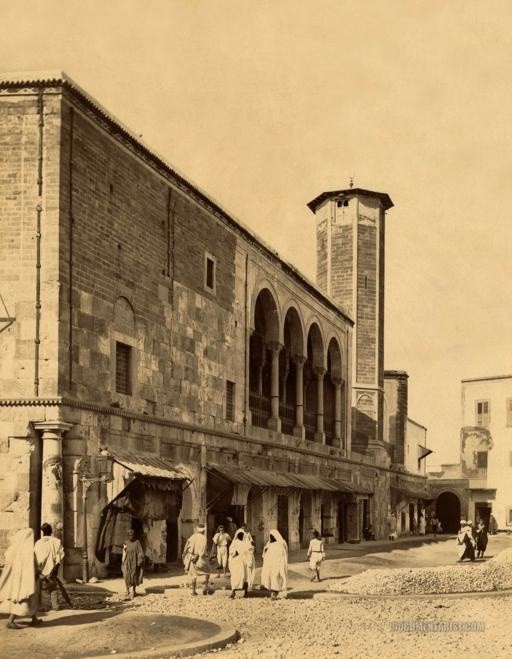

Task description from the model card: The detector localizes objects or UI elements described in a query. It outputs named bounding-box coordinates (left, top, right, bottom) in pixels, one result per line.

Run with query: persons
left=474, top=517, right=489, bottom=559
left=210, top=524, right=233, bottom=579
left=228, top=531, right=256, bottom=600
left=305, top=529, right=326, bottom=582
left=239, top=523, right=254, bottom=547
left=489, top=513, right=498, bottom=536
left=260, top=529, right=289, bottom=601
left=418, top=512, right=427, bottom=537
left=119, top=529, right=146, bottom=603
left=456, top=520, right=477, bottom=563
left=364, top=524, right=376, bottom=542
left=387, top=512, right=398, bottom=541
left=430, top=516, right=440, bottom=537
left=0, top=527, right=43, bottom=629
left=180, top=523, right=216, bottom=596
left=33, top=520, right=65, bottom=614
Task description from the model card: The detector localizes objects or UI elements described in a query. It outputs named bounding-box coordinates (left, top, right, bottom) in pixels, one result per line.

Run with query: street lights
left=78, top=447, right=114, bottom=585
